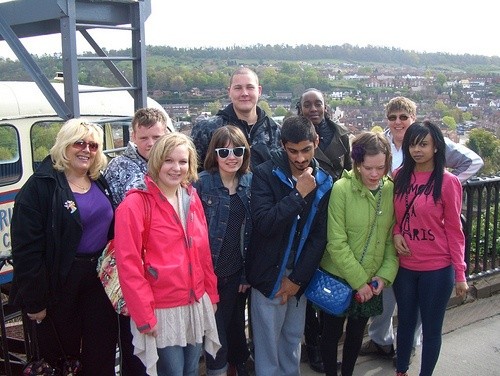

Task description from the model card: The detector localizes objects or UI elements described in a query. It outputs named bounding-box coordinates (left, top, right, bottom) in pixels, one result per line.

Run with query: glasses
left=72, top=139, right=98, bottom=152
left=388, top=114, right=411, bottom=121
left=215, top=147, right=246, bottom=159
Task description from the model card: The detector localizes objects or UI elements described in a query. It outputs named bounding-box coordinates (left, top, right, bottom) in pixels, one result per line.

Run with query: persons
left=358, top=97, right=484, bottom=367
left=102, top=108, right=169, bottom=376
left=10, top=119, right=120, bottom=376
left=191, top=68, right=284, bottom=376
left=245, top=116, right=334, bottom=376
left=391, top=120, right=469, bottom=376
left=115, top=132, right=219, bottom=376
left=319, top=134, right=400, bottom=376
left=295, top=88, right=352, bottom=374
left=189, top=125, right=254, bottom=376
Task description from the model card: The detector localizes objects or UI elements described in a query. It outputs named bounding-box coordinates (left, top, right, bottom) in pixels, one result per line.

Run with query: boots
left=305, top=317, right=341, bottom=373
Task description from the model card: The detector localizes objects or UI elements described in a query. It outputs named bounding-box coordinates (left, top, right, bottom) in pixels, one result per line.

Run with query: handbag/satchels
left=22, top=315, right=83, bottom=376
left=304, top=269, right=353, bottom=316
left=96, top=192, right=152, bottom=316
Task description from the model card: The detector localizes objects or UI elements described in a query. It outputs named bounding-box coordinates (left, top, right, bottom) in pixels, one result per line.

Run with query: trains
left=0, top=71, right=174, bottom=322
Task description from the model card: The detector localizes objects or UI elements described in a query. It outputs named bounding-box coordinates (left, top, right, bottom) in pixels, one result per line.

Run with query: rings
left=37, top=320, right=42, bottom=324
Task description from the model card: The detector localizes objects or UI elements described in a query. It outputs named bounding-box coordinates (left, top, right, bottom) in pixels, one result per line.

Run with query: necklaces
left=66, top=176, right=92, bottom=190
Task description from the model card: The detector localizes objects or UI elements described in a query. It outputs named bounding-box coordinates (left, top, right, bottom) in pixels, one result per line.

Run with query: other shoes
left=236, top=360, right=248, bottom=376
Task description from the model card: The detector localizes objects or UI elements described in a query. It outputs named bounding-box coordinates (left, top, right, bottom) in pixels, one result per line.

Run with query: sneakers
left=359, top=339, right=395, bottom=359
left=393, top=347, right=416, bottom=371
